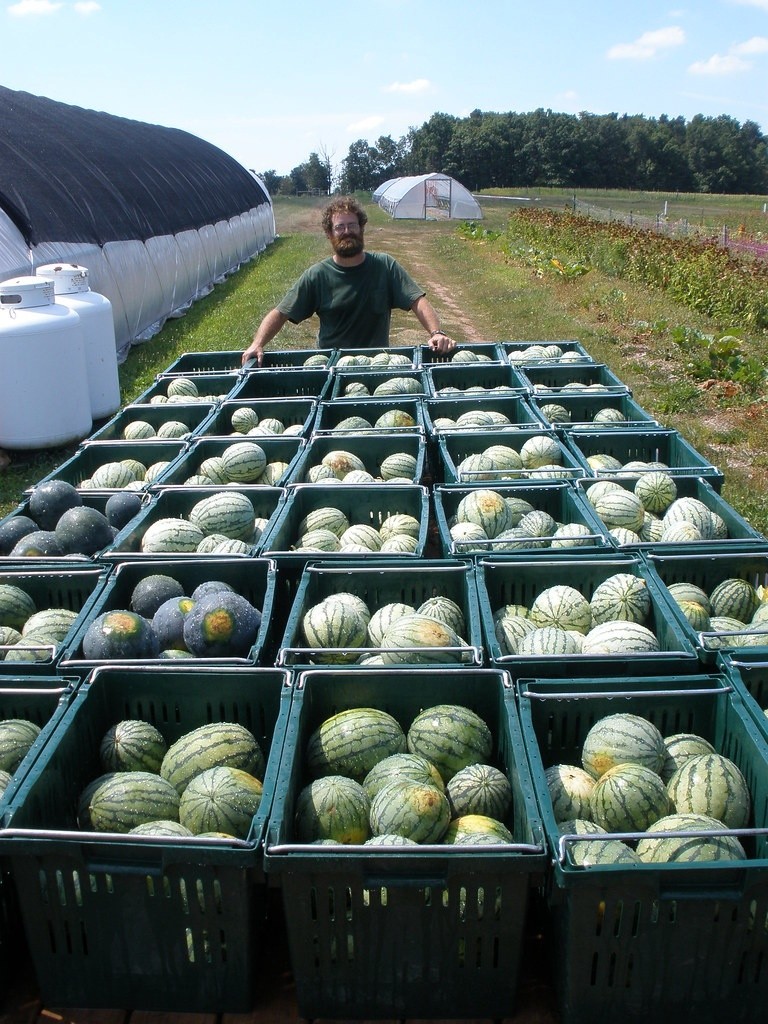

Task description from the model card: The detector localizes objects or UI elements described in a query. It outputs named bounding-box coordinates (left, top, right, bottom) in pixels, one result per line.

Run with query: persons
left=241, top=195, right=458, bottom=368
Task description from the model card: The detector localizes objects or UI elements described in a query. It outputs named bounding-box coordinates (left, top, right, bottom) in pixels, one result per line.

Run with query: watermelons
left=0, top=343, right=768, bottom=968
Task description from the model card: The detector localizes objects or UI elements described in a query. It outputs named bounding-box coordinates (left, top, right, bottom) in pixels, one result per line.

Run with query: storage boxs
left=0, top=340, right=768, bottom=570
left=56, top=557, right=279, bottom=681
left=715, top=646, right=768, bottom=747
left=637, top=544, right=768, bottom=675
left=0, top=562, right=114, bottom=676
left=514, top=674, right=768, bottom=1024
left=0, top=674, right=84, bottom=1020
left=473, top=554, right=700, bottom=684
left=260, top=668, right=549, bottom=1020
left=0, top=665, right=298, bottom=1015
left=273, top=558, right=485, bottom=683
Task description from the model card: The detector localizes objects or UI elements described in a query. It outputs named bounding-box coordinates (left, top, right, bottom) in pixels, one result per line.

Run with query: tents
left=372, top=172, right=484, bottom=221
left=0, top=87, right=278, bottom=367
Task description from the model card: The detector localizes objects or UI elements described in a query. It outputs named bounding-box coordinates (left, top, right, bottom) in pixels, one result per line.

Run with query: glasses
left=331, top=224, right=360, bottom=233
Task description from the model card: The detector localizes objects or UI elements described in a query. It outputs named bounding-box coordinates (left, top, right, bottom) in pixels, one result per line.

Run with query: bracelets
left=431, top=329, right=446, bottom=338
